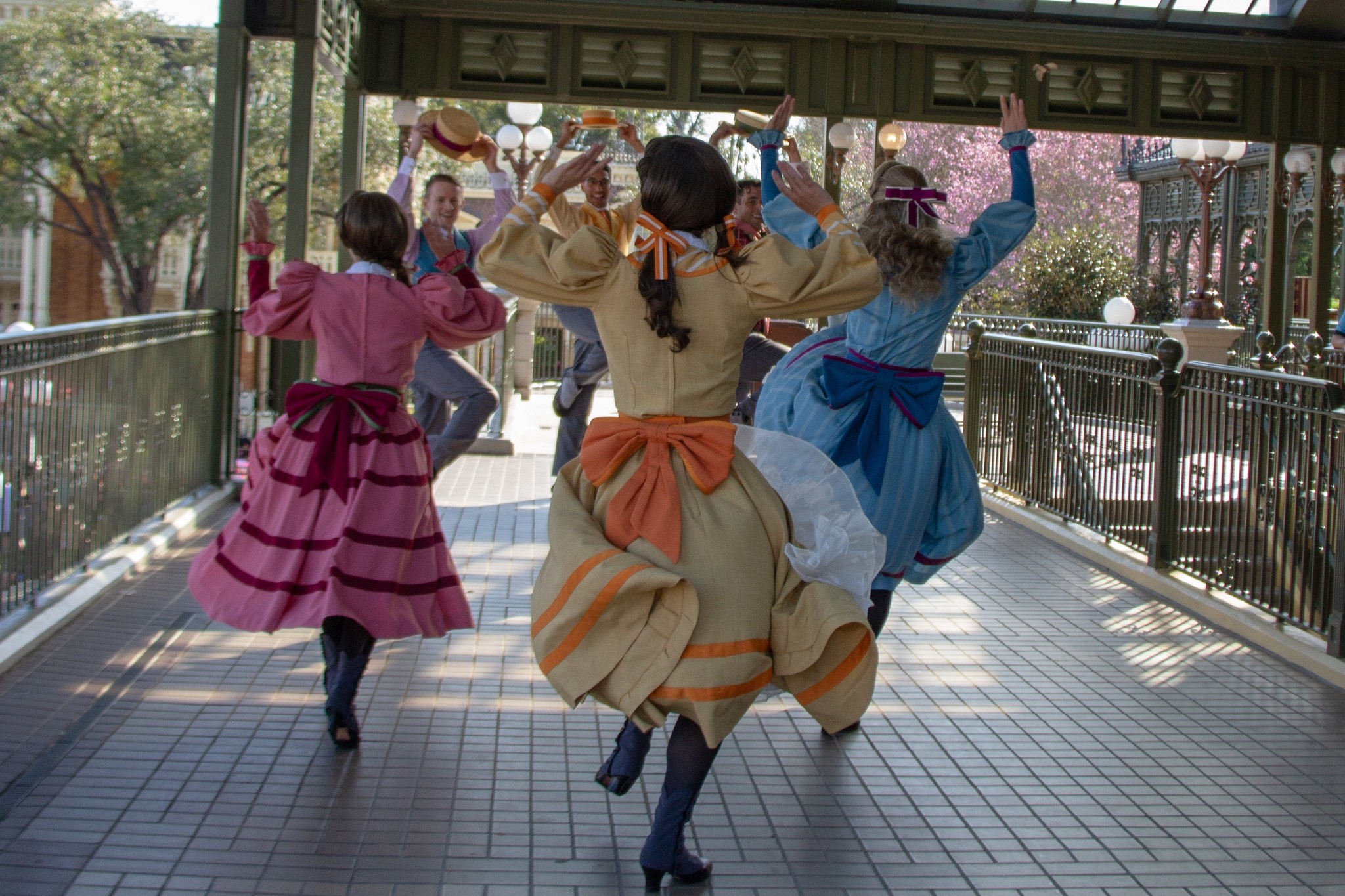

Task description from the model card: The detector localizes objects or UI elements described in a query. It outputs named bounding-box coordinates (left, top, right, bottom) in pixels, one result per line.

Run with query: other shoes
left=821, top=719, right=860, bottom=740
left=552, top=374, right=583, bottom=418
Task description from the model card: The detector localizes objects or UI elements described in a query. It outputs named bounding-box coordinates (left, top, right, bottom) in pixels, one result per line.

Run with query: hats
left=570, top=109, right=628, bottom=130
left=717, top=109, right=770, bottom=137
left=418, top=107, right=490, bottom=163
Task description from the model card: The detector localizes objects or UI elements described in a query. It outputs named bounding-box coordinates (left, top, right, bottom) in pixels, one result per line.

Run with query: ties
left=447, top=234, right=454, bottom=241
left=598, top=210, right=612, bottom=234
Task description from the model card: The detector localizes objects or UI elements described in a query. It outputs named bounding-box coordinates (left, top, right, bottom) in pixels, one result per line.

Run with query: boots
left=595, top=717, right=652, bottom=795
left=320, top=633, right=366, bottom=749
left=640, top=785, right=713, bottom=894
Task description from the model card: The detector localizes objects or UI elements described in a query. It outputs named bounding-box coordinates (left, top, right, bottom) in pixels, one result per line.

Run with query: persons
left=532, top=119, right=647, bottom=491
left=750, top=92, right=1039, bottom=728
left=475, top=142, right=887, bottom=891
left=708, top=123, right=811, bottom=424
left=191, top=190, right=508, bottom=748
left=387, top=122, right=517, bottom=481
